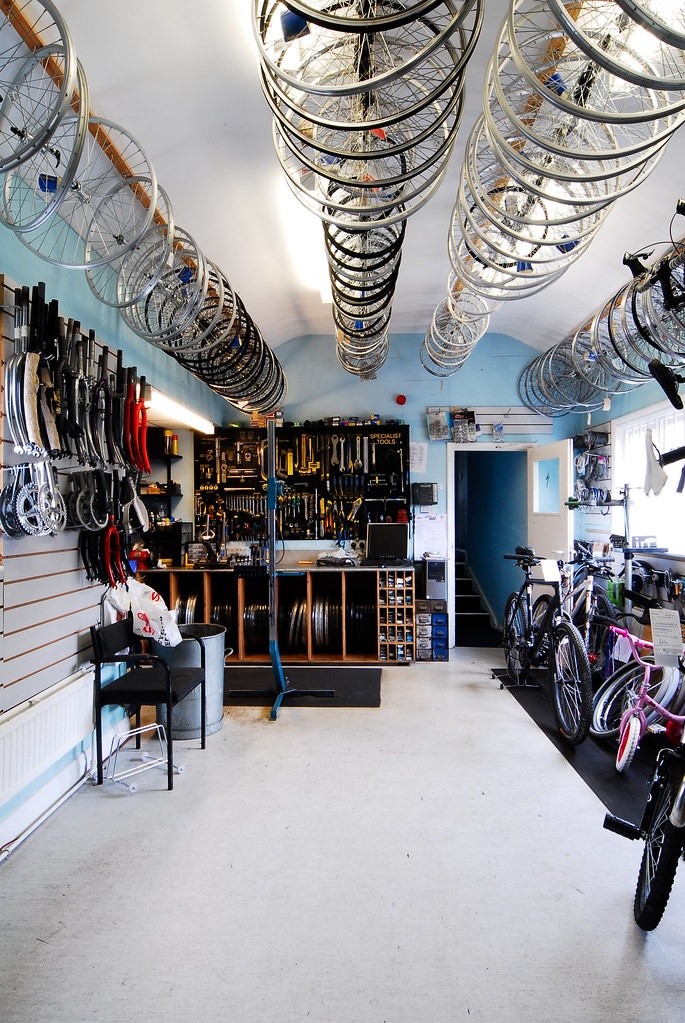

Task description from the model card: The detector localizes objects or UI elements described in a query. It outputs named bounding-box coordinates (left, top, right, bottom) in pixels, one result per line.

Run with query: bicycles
left=488, top=536, right=685, bottom=933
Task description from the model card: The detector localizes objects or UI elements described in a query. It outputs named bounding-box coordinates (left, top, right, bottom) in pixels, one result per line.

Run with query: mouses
left=378, top=564, right=387, bottom=568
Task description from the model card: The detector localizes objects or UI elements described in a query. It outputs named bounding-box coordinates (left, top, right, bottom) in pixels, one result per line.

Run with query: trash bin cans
left=151, top=623, right=227, bottom=741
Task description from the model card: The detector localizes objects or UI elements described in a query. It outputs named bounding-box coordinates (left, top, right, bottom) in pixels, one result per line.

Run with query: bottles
left=172, top=435, right=178, bottom=455
left=164, top=430, right=172, bottom=454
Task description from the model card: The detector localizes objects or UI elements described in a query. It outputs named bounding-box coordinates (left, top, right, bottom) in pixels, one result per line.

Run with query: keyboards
left=360, top=559, right=404, bottom=567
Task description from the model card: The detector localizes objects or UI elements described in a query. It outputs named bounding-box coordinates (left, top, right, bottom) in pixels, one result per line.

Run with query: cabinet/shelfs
left=133, top=562, right=418, bottom=665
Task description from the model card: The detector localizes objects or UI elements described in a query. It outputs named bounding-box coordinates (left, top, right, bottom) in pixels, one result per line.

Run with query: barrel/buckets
left=150, top=623, right=233, bottom=739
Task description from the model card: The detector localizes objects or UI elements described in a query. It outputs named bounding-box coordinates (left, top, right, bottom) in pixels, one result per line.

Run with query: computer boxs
left=422, top=557, right=446, bottom=600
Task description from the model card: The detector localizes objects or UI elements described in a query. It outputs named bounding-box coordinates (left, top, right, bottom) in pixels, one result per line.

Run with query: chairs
left=87, top=610, right=206, bottom=791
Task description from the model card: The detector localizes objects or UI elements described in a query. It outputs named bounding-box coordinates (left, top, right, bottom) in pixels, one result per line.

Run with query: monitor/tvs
left=366, top=522, right=409, bottom=560
